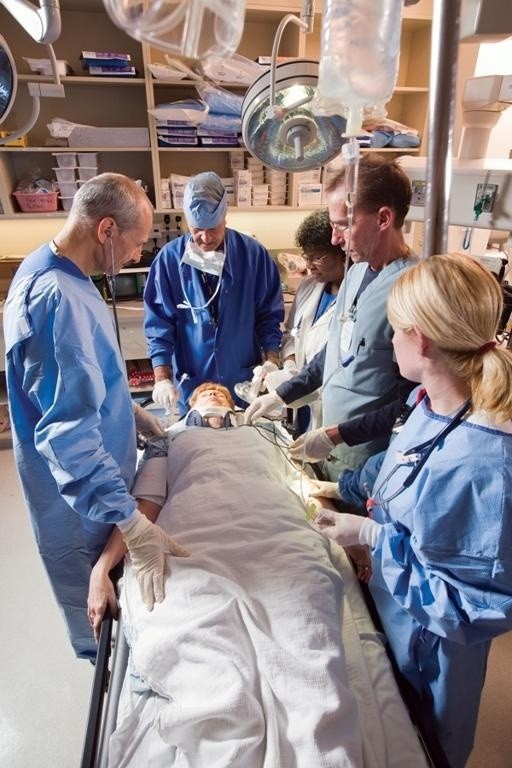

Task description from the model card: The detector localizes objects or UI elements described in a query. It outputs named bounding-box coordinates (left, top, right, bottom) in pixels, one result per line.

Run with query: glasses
left=301, top=253, right=326, bottom=265
left=329, top=220, right=347, bottom=231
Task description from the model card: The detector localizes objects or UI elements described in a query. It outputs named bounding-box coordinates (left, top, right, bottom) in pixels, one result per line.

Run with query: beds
left=80, top=396, right=449, bottom=767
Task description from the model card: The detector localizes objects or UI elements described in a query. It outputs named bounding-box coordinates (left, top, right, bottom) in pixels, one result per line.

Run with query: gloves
left=152, top=379, right=179, bottom=409
left=287, top=427, right=335, bottom=463
left=309, top=479, right=338, bottom=498
left=136, top=407, right=164, bottom=437
left=284, top=359, right=296, bottom=371
left=115, top=509, right=191, bottom=612
left=326, top=513, right=381, bottom=548
left=243, top=360, right=282, bottom=425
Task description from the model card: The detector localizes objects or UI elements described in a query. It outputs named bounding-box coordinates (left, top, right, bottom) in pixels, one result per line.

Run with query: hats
left=183, top=171, right=227, bottom=229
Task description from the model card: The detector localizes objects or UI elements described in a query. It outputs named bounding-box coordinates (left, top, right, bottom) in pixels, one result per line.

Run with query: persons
left=309, top=252, right=512, bottom=768
left=144, top=171, right=285, bottom=411
left=89, top=385, right=355, bottom=768
left=266, top=206, right=353, bottom=438
left=3, top=172, right=190, bottom=665
left=244, top=154, right=421, bottom=510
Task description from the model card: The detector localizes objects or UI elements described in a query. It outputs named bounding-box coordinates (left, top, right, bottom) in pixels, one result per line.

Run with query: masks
left=181, top=240, right=225, bottom=276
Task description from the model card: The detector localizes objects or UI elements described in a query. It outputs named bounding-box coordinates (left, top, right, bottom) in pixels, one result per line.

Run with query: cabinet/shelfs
left=0, top=0, right=433, bottom=219
